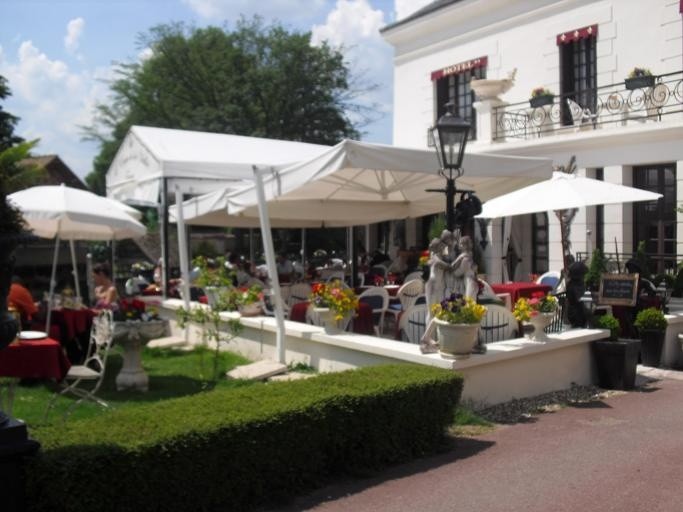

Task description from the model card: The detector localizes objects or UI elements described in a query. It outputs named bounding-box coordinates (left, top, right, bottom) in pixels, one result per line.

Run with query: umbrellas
left=67, top=192, right=141, bottom=304
left=472, top=171, right=663, bottom=276
left=6, top=183, right=144, bottom=337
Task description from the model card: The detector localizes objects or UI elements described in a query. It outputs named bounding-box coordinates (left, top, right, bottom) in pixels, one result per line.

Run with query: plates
left=16, top=330, right=49, bottom=340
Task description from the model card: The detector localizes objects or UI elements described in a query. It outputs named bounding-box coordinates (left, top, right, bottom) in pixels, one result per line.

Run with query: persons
left=621, top=252, right=655, bottom=338
left=125, top=236, right=416, bottom=311
left=553, top=254, right=572, bottom=289
left=4, top=273, right=37, bottom=325
left=563, top=262, right=591, bottom=327
left=420, top=227, right=483, bottom=355
left=67, top=263, right=118, bottom=366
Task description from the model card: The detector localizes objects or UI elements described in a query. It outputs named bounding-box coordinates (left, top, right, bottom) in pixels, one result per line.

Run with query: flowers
left=628, top=65, right=651, bottom=77
left=532, top=87, right=550, bottom=97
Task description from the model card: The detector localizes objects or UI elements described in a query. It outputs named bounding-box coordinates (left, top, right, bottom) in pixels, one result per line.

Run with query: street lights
left=423, top=101, right=474, bottom=230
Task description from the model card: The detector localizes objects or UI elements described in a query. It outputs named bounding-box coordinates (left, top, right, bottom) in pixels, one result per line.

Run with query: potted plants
left=595, top=315, right=641, bottom=390
left=635, top=308, right=670, bottom=366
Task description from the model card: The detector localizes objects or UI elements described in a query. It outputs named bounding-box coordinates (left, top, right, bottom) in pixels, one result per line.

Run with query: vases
left=625, top=75, right=655, bottom=89
left=529, top=94, right=555, bottom=107
